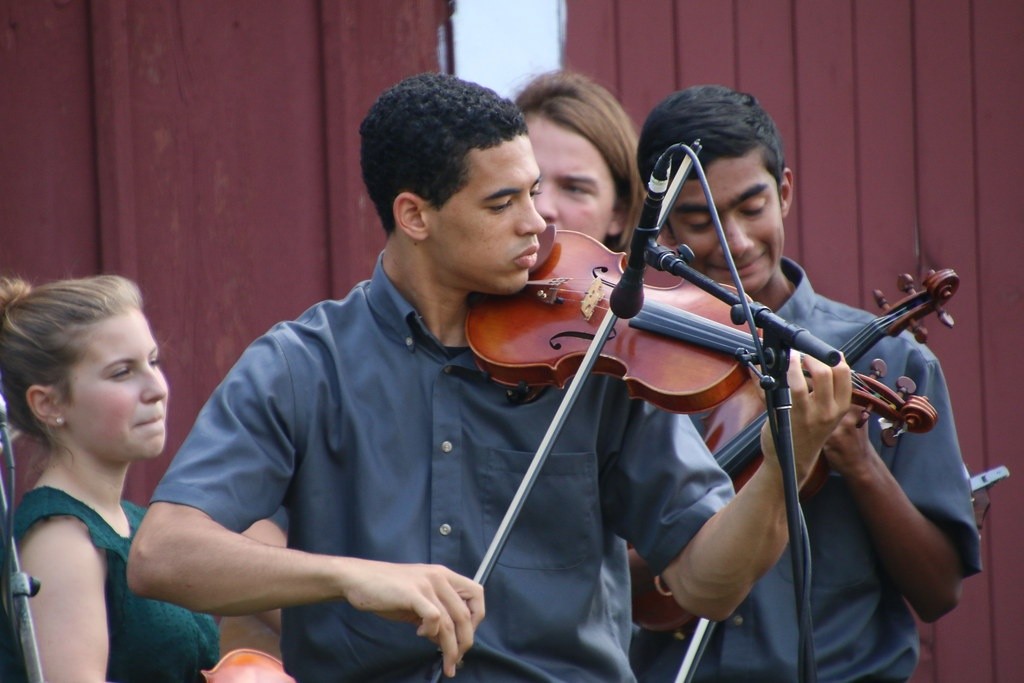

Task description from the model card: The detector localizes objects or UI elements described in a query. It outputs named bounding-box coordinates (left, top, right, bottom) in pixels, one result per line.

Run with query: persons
left=239, top=70, right=650, bottom=639
left=0, top=275, right=221, bottom=683
left=626, top=82, right=984, bottom=683
left=127, top=72, right=851, bottom=683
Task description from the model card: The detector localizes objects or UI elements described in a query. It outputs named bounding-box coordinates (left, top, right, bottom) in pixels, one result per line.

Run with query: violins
left=462, top=226, right=941, bottom=450
left=200, top=645, right=298, bottom=683
left=626, top=265, right=962, bottom=643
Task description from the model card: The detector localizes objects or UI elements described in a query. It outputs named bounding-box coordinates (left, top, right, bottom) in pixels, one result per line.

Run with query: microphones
left=610, top=153, right=673, bottom=319
